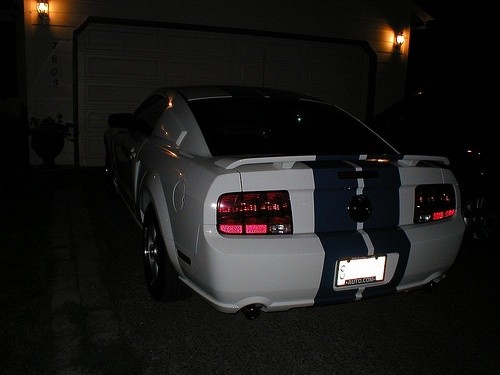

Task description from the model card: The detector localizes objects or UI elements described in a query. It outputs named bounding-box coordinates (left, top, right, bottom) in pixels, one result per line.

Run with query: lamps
left=393, top=29, right=404, bottom=54
left=37, top=0, right=50, bottom=30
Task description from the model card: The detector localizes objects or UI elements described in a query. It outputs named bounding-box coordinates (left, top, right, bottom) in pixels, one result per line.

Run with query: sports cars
left=100, top=82, right=467, bottom=321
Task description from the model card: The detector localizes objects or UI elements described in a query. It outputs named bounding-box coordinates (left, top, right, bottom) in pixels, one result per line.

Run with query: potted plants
left=8, top=113, right=80, bottom=164
